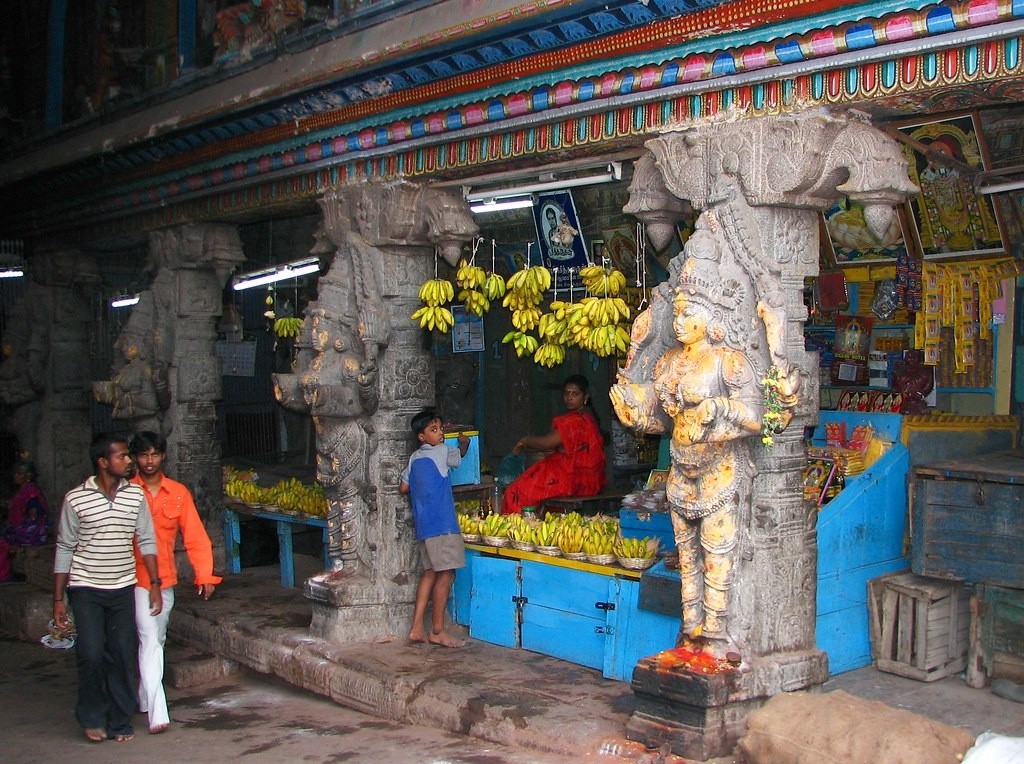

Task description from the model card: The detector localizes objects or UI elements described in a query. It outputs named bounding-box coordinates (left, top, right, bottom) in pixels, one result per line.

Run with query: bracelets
left=53, top=599, right=62, bottom=601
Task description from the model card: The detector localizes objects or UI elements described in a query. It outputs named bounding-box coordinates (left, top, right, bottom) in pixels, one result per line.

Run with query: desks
left=448, top=541, right=681, bottom=684
left=453, top=484, right=494, bottom=501
left=222, top=498, right=333, bottom=589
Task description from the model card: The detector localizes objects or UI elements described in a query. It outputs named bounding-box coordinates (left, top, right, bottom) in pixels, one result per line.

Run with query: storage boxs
left=445, top=431, right=480, bottom=487
left=910, top=449, right=1024, bottom=589
left=966, top=583, right=1024, bottom=690
left=620, top=469, right=679, bottom=558
left=867, top=569, right=972, bottom=683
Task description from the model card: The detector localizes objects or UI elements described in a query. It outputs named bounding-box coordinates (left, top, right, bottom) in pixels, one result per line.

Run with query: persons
left=501, top=375, right=606, bottom=514
left=892, top=349, right=933, bottom=416
left=130, top=431, right=222, bottom=733
left=0, top=335, right=42, bottom=461
left=514, top=254, right=525, bottom=273
left=400, top=412, right=471, bottom=648
left=51, top=432, right=163, bottom=742
left=609, top=271, right=759, bottom=673
left=546, top=207, right=567, bottom=249
left=90, top=336, right=162, bottom=439
left=0, top=461, right=50, bottom=582
left=272, top=304, right=379, bottom=584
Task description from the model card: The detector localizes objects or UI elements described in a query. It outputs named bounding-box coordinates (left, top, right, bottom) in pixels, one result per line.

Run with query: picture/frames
left=818, top=196, right=914, bottom=269
left=884, top=110, right=1011, bottom=263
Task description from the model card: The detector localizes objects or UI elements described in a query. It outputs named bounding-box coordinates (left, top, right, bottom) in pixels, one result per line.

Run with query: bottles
left=809, top=450, right=845, bottom=504
left=489, top=477, right=502, bottom=515
left=522, top=506, right=536, bottom=527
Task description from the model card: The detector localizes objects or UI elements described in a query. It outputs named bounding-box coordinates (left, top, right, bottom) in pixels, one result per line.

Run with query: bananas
left=273, top=316, right=303, bottom=338
left=455, top=511, right=657, bottom=560
left=409, top=251, right=630, bottom=369
left=222, top=464, right=333, bottom=515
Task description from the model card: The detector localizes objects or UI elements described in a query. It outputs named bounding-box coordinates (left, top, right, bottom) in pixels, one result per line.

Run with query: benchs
left=537, top=492, right=625, bottom=521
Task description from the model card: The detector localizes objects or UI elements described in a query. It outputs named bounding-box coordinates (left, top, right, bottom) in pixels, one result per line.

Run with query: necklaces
left=148, top=486, right=154, bottom=491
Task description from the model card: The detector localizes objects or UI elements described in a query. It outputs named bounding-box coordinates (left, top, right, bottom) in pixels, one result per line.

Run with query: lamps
left=470, top=193, right=540, bottom=214
left=461, top=161, right=623, bottom=203
left=973, top=165, right=1024, bottom=194
left=233, top=255, right=327, bottom=290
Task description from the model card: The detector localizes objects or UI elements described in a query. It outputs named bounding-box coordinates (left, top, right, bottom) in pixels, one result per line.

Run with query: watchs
left=151, top=578, right=162, bottom=585
